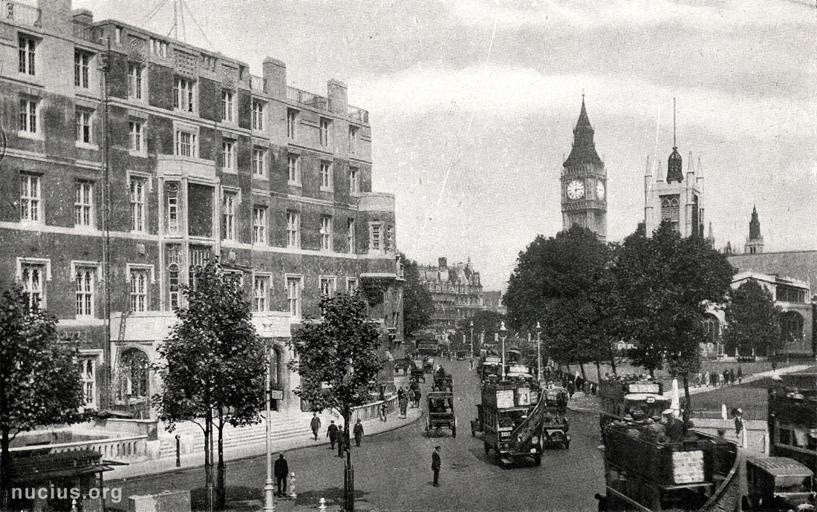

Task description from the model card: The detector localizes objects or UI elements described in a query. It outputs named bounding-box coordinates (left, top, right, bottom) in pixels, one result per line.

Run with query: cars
left=411, top=367, right=426, bottom=384
left=543, top=382, right=570, bottom=406
left=476, top=344, right=548, bottom=381
left=542, top=414, right=571, bottom=449
left=739, top=456, right=817, bottom=512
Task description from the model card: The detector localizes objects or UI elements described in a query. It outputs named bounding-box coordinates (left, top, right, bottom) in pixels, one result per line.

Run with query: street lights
left=259, top=320, right=274, bottom=512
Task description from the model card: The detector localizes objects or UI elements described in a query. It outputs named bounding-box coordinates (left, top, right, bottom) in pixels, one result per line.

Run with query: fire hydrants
left=317, top=497, right=327, bottom=512
left=287, top=471, right=297, bottom=499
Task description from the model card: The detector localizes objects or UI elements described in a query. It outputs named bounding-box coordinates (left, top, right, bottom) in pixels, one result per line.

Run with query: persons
left=335, top=424, right=346, bottom=458
left=308, top=412, right=321, bottom=442
left=326, top=419, right=337, bottom=450
left=379, top=343, right=452, bottom=422
left=273, top=452, right=289, bottom=498
left=469, top=348, right=757, bottom=452
left=772, top=360, right=778, bottom=372
left=430, top=444, right=442, bottom=487
left=352, top=418, right=364, bottom=448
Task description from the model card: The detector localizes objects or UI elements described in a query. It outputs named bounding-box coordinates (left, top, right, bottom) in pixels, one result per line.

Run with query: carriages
left=425, top=364, right=456, bottom=439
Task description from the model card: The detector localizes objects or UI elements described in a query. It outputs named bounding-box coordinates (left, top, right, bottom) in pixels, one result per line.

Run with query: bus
left=470, top=374, right=544, bottom=469
left=768, top=389, right=817, bottom=490
left=596, top=376, right=742, bottom=512
left=418, top=341, right=446, bottom=356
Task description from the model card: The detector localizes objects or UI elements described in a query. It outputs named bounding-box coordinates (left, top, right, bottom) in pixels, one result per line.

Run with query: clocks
left=597, top=180, right=605, bottom=201
left=566, top=181, right=584, bottom=200
